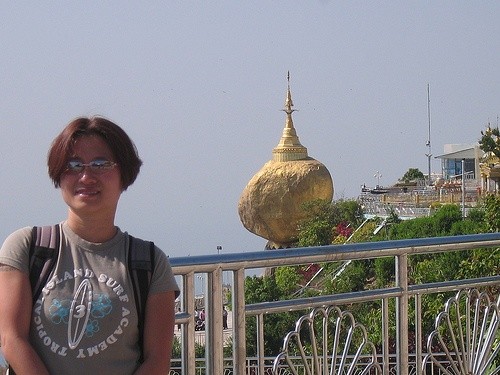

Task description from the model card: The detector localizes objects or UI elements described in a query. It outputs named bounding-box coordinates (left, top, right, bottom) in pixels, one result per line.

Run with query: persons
left=0, top=115, right=180, bottom=375
left=178, top=306, right=181, bottom=331
left=194, top=305, right=205, bottom=330
left=222, top=306, right=229, bottom=330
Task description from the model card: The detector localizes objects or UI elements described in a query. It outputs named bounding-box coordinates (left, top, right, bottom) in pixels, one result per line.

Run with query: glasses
left=62, top=160, right=120, bottom=174
